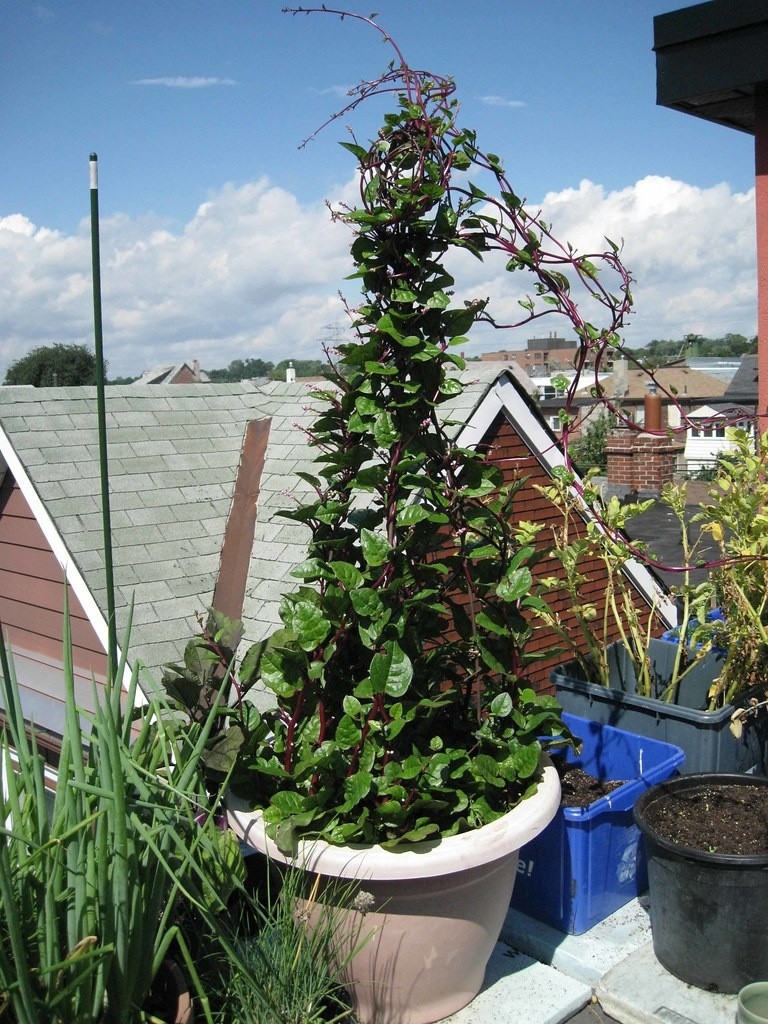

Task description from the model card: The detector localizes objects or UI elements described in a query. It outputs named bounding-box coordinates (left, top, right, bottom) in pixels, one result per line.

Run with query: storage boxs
left=510, top=705, right=685, bottom=935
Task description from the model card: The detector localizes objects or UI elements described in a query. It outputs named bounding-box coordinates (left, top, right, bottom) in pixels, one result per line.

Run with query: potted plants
left=536, top=396, right=768, bottom=771
left=226, top=5, right=587, bottom=1024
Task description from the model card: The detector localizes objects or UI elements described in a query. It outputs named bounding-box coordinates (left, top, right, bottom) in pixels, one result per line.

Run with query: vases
left=634, top=768, right=768, bottom=991
